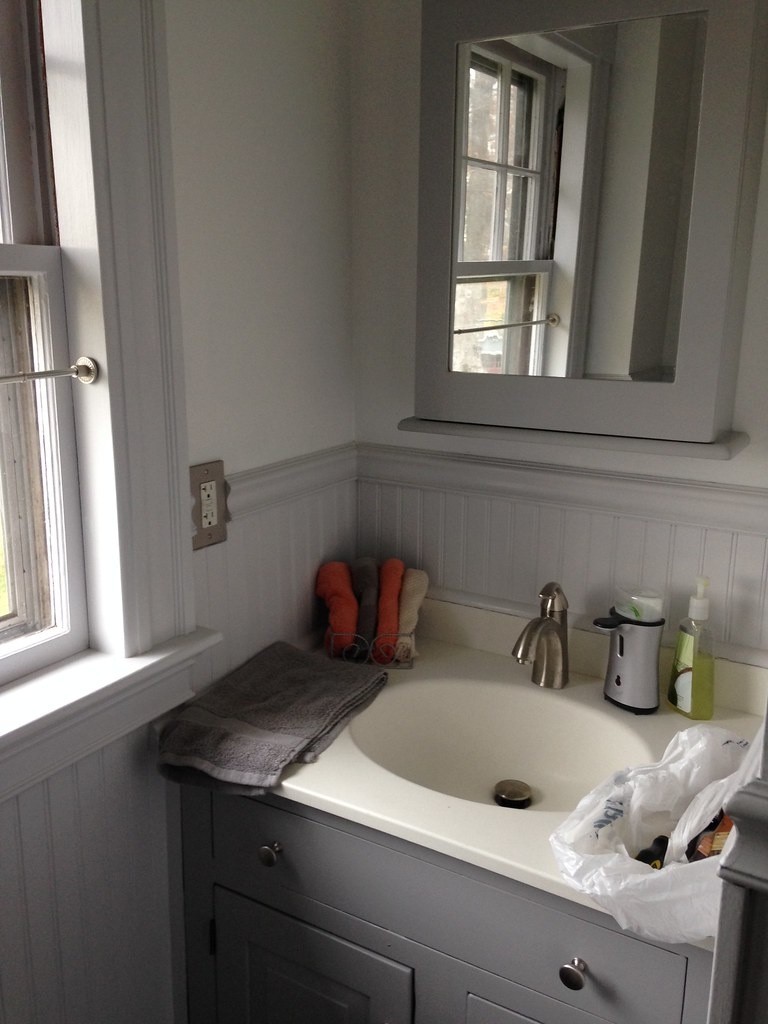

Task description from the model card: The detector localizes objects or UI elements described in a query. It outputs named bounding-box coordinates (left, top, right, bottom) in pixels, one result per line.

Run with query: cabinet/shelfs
left=159, top=758, right=768, bottom=1024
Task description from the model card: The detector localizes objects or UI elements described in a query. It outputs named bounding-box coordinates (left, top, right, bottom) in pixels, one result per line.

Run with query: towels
left=313, top=556, right=429, bottom=665
left=155, top=639, right=390, bottom=789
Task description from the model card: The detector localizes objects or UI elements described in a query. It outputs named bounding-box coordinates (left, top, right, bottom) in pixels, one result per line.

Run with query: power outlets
left=190, top=459, right=227, bottom=551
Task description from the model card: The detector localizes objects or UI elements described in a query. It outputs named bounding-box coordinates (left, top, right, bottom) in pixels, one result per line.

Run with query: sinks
left=347, top=677, right=654, bottom=813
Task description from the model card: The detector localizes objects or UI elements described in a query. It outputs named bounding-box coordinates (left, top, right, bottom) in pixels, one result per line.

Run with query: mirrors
left=414, top=0, right=767, bottom=445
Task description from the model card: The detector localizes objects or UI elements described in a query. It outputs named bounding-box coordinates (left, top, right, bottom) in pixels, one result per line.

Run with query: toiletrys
left=592, top=581, right=667, bottom=716
left=666, top=576, right=715, bottom=721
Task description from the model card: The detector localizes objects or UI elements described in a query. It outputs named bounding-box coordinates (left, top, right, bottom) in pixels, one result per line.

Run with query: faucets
left=511, top=581, right=569, bottom=689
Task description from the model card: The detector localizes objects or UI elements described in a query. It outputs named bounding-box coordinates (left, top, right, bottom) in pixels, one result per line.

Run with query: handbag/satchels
left=550, top=724, right=750, bottom=944
